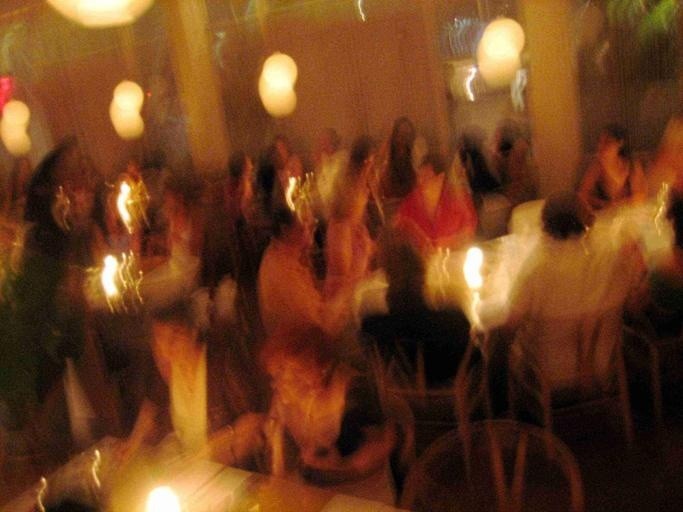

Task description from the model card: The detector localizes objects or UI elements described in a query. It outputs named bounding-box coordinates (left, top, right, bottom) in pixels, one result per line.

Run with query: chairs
left=369, top=314, right=639, bottom=511
